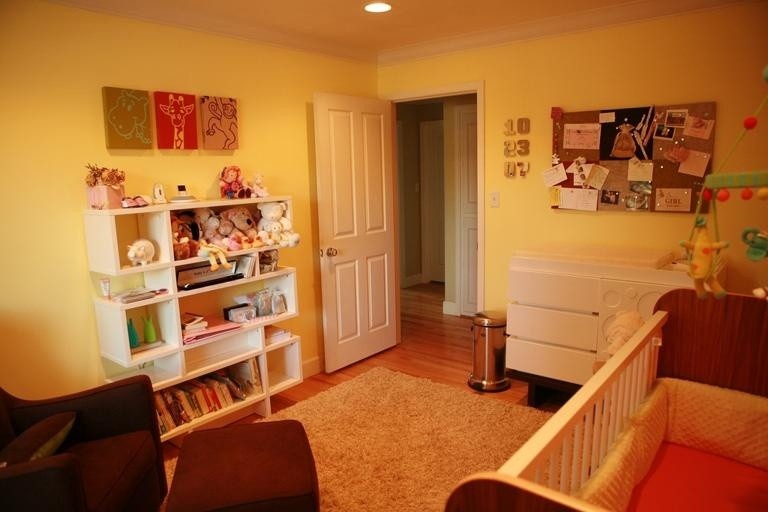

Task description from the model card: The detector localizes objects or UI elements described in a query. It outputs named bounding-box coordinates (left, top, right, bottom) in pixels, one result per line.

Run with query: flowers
left=85, top=164, right=125, bottom=191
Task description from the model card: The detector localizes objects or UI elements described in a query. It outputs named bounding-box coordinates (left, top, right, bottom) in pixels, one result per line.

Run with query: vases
left=87, top=186, right=123, bottom=209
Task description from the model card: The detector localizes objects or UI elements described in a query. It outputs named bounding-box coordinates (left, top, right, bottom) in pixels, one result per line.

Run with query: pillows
left=0, top=411, right=77, bottom=467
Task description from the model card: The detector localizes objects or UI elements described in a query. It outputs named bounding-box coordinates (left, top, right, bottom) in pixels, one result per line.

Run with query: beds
left=444, top=288, right=768, bottom=512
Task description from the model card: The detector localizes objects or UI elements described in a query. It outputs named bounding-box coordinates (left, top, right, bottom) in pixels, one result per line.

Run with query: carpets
left=158, top=366, right=602, bottom=512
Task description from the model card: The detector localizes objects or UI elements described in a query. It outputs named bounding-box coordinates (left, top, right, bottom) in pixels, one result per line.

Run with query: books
left=181, top=311, right=209, bottom=333
left=114, top=286, right=155, bottom=303
left=153, top=355, right=263, bottom=435
left=264, top=325, right=291, bottom=346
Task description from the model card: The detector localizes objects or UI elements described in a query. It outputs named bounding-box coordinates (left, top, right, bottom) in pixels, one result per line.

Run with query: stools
left=167, top=418, right=320, bottom=512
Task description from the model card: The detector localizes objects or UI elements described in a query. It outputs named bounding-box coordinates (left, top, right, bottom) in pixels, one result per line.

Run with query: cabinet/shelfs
left=83, top=195, right=304, bottom=449
left=504, top=258, right=696, bottom=408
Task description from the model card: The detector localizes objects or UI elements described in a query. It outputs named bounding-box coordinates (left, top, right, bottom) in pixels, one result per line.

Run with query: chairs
left=0, top=374, right=168, bottom=512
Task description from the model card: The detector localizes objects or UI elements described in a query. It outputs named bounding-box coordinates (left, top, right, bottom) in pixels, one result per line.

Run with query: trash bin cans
left=467, top=311, right=511, bottom=391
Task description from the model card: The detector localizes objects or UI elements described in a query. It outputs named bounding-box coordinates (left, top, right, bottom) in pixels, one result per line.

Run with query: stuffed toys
left=219, top=165, right=251, bottom=199
left=251, top=172, right=271, bottom=198
left=673, top=66, right=768, bottom=300
left=170, top=201, right=301, bottom=271
